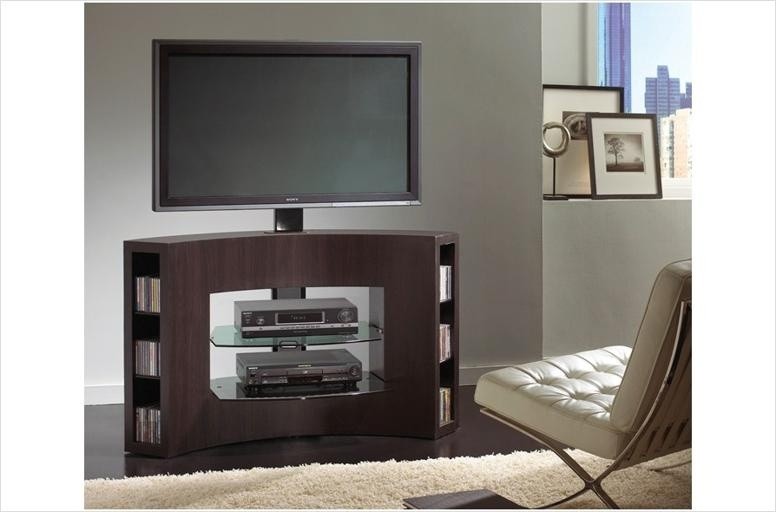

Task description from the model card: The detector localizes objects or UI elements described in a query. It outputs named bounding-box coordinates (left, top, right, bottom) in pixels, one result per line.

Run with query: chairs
left=473, top=258, right=691, bottom=509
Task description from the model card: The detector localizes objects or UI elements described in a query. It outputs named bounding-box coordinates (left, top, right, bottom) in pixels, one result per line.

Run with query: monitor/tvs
left=152, top=37, right=423, bottom=232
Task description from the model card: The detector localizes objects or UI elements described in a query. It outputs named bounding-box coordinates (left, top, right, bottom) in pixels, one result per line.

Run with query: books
left=403, top=488, right=530, bottom=510
left=438, top=384, right=451, bottom=424
left=439, top=321, right=451, bottom=363
left=134, top=275, right=160, bottom=313
left=134, top=403, right=161, bottom=446
left=133, top=339, right=160, bottom=377
left=438, top=265, right=452, bottom=302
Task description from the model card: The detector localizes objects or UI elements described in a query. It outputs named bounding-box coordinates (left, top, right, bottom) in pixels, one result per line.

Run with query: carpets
left=84, top=448, right=691, bottom=509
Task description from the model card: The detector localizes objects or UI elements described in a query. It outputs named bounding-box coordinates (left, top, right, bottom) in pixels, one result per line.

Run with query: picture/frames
left=541, top=84, right=625, bottom=199
left=584, top=112, right=663, bottom=200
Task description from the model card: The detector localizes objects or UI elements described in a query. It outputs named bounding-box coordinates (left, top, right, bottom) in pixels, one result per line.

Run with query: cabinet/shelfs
left=123, top=229, right=461, bottom=456
